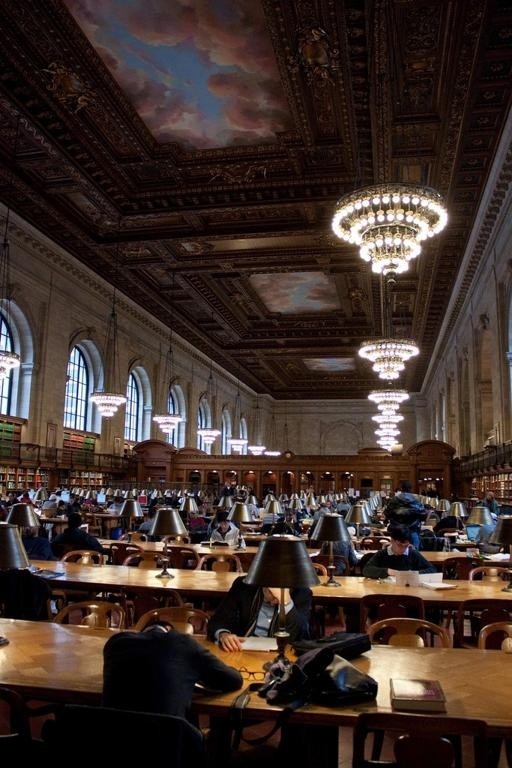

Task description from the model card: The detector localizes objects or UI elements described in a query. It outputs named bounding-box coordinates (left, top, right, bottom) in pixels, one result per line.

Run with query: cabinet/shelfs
left=471, top=471, right=512, bottom=504
left=58, top=464, right=113, bottom=491
left=0, top=461, right=49, bottom=493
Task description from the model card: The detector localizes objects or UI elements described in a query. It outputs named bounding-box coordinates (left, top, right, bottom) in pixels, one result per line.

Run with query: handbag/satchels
left=291, top=654, right=377, bottom=707
left=289, top=633, right=371, bottom=659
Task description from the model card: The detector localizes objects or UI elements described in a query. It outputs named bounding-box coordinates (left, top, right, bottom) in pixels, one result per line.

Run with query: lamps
left=152, top=269, right=183, bottom=433
left=0, top=166, right=22, bottom=378
left=332, top=1, right=449, bottom=276
left=226, top=358, right=282, bottom=458
left=89, top=209, right=129, bottom=418
left=196, top=311, right=222, bottom=445
left=367, top=389, right=410, bottom=452
left=357, top=337, right=420, bottom=381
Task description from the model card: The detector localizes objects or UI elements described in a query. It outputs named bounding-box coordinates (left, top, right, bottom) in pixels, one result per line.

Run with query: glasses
left=395, top=542, right=409, bottom=547
left=239, top=666, right=267, bottom=681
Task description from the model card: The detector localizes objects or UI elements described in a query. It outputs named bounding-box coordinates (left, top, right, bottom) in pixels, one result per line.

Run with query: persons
left=103, top=619, right=243, bottom=730
left=0, top=482, right=512, bottom=651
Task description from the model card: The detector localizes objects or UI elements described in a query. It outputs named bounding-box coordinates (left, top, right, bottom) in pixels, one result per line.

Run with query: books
left=467, top=467, right=512, bottom=502
left=0, top=466, right=105, bottom=494
left=390, top=676, right=448, bottom=712
